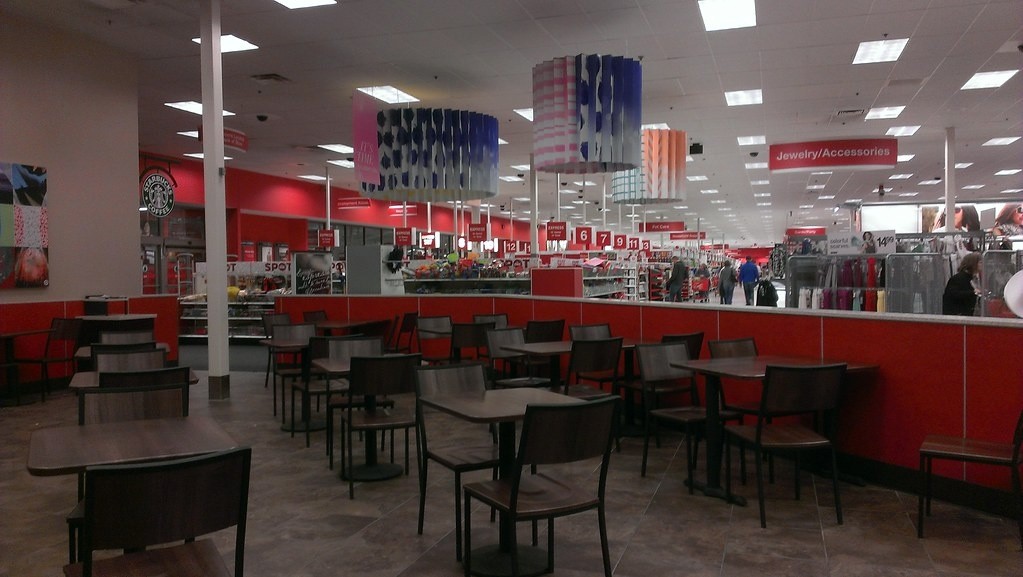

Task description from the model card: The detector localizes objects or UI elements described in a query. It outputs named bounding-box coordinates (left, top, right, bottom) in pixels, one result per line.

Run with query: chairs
left=917, top=408, right=1023, bottom=539
left=443, top=320, right=497, bottom=382
left=463, top=393, right=626, bottom=577
left=511, top=317, right=568, bottom=383
left=337, top=352, right=419, bottom=497
left=18, top=318, right=192, bottom=392
left=66, top=380, right=188, bottom=565
left=413, top=363, right=539, bottom=538
left=260, top=309, right=365, bottom=448
left=473, top=311, right=511, bottom=368
left=615, top=330, right=706, bottom=446
left=564, top=323, right=622, bottom=393
left=382, top=311, right=420, bottom=355
left=372, top=315, right=400, bottom=350
left=545, top=336, right=625, bottom=447
left=633, top=341, right=748, bottom=493
left=415, top=315, right=468, bottom=368
left=485, top=327, right=552, bottom=437
left=62, top=446, right=255, bottom=577
left=727, top=362, right=847, bottom=530
left=707, top=336, right=796, bottom=486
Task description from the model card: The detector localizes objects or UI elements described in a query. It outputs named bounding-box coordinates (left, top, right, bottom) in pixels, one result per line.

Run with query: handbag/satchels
left=731, top=268, right=737, bottom=282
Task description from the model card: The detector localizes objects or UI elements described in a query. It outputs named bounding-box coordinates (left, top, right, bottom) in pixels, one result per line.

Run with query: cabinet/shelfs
left=404, top=248, right=742, bottom=297
left=178, top=286, right=288, bottom=339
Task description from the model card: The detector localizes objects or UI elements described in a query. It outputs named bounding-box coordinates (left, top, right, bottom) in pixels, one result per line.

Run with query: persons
left=717, top=262, right=728, bottom=304
left=857, top=231, right=878, bottom=254
left=929, top=204, right=981, bottom=233
left=719, top=259, right=738, bottom=305
left=990, top=202, right=1023, bottom=237
left=739, top=256, right=760, bottom=306
left=665, top=256, right=686, bottom=302
left=750, top=259, right=762, bottom=277
left=940, top=252, right=983, bottom=316
left=695, top=263, right=710, bottom=303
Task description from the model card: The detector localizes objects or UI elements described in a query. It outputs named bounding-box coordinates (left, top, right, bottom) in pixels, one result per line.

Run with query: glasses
left=944, top=207, right=963, bottom=214
left=1017, top=207, right=1023, bottom=214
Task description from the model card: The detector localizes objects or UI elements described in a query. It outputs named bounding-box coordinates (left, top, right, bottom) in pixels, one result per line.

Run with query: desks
left=72, top=341, right=171, bottom=368
left=69, top=367, right=201, bottom=425
left=309, top=316, right=375, bottom=342
left=669, top=354, right=881, bottom=508
left=500, top=340, right=648, bottom=384
left=419, top=387, right=590, bottom=577
left=427, top=325, right=505, bottom=368
left=262, top=338, right=339, bottom=431
left=27, top=414, right=239, bottom=557
left=0, top=328, right=60, bottom=408
left=312, top=355, right=422, bottom=480
